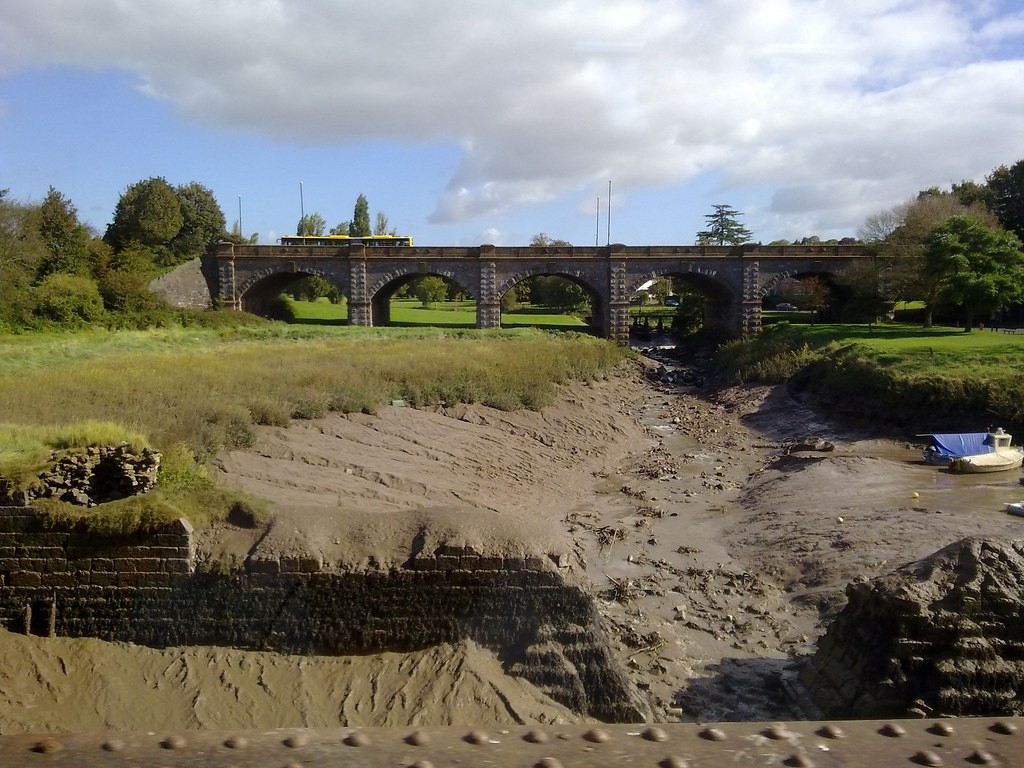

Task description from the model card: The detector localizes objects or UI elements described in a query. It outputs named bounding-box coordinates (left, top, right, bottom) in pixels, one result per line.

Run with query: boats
left=920, top=431, right=996, bottom=464
left=948, top=448, right=1024, bottom=474
left=1003, top=501, right=1024, bottom=517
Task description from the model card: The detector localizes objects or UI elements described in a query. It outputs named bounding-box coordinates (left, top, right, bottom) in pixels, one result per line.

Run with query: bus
left=274, top=233, right=414, bottom=247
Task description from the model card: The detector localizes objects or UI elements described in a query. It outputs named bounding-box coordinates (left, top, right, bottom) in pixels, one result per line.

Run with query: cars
left=776, top=303, right=798, bottom=311
left=629, top=298, right=643, bottom=305
left=664, top=299, right=680, bottom=307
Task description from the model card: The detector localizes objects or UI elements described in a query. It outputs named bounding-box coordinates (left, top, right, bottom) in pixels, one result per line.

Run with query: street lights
left=237, top=194, right=242, bottom=237
left=299, top=181, right=305, bottom=233
